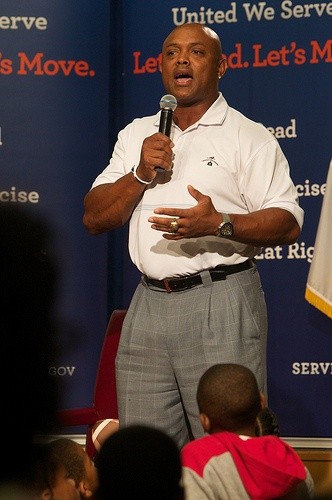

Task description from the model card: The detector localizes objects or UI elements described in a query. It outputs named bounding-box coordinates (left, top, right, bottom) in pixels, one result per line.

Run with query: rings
left=170, top=218, right=178, bottom=231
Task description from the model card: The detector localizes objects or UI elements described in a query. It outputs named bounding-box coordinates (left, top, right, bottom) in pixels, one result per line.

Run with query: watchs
left=214, top=213, right=233, bottom=238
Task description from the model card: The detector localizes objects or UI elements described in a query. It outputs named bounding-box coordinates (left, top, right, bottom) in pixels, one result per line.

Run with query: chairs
left=58, top=309, right=127, bottom=462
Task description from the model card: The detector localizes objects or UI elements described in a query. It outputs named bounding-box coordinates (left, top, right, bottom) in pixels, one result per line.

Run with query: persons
left=39, top=424, right=187, bottom=500
left=181, top=363, right=315, bottom=500
left=83, top=23, right=304, bottom=450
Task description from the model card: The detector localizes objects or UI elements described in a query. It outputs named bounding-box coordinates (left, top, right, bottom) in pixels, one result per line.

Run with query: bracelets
left=131, top=165, right=153, bottom=185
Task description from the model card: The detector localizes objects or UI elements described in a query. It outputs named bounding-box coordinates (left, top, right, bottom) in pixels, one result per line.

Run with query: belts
left=145, top=258, right=253, bottom=292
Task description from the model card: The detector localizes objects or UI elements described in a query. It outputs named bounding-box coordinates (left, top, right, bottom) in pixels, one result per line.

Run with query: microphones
left=155, top=94, right=178, bottom=173
